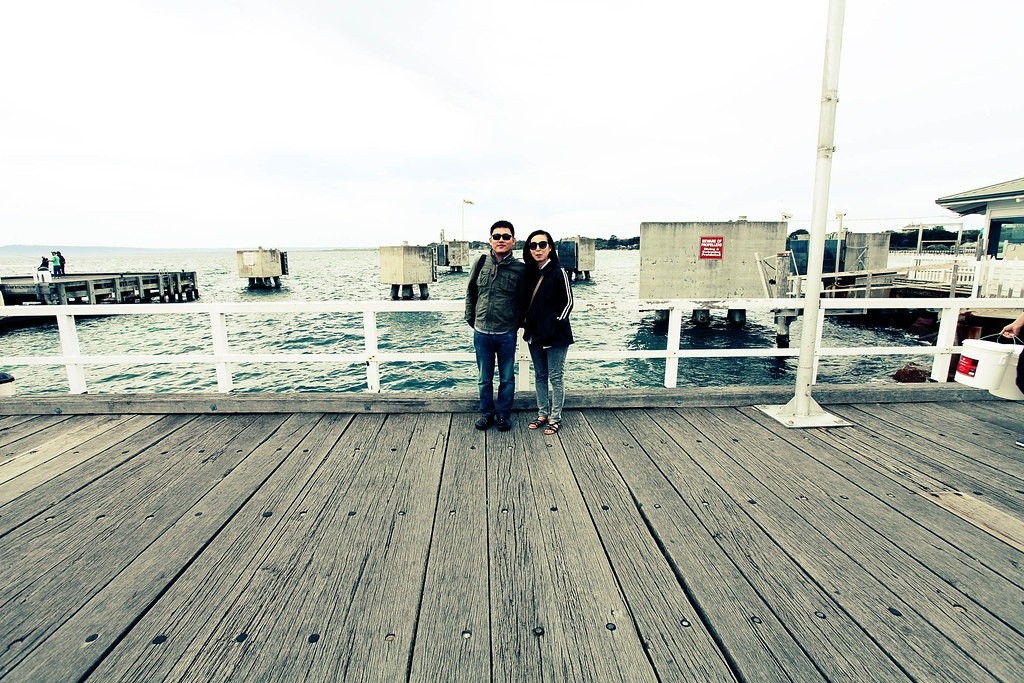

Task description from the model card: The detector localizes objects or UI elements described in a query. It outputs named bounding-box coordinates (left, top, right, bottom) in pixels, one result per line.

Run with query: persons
left=513, top=228, right=576, bottom=435
left=56, top=251, right=66, bottom=277
left=47, top=251, right=61, bottom=279
left=996, top=303, right=1024, bottom=449
left=464, top=219, right=529, bottom=431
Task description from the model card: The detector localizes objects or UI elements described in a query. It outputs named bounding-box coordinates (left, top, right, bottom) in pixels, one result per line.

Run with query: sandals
left=528, top=415, right=551, bottom=430
left=545, top=420, right=562, bottom=435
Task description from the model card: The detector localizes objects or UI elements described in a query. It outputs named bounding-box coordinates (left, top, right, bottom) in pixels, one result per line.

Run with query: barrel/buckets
left=988, top=333, right=1024, bottom=401
left=953, top=332, right=1015, bottom=390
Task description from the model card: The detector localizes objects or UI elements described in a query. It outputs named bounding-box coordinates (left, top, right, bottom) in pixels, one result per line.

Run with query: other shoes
left=497, top=417, right=512, bottom=430
left=475, top=416, right=495, bottom=430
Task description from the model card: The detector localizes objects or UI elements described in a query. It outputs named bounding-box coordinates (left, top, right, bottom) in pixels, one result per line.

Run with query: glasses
left=490, top=233, right=513, bottom=240
left=529, top=241, right=550, bottom=250
